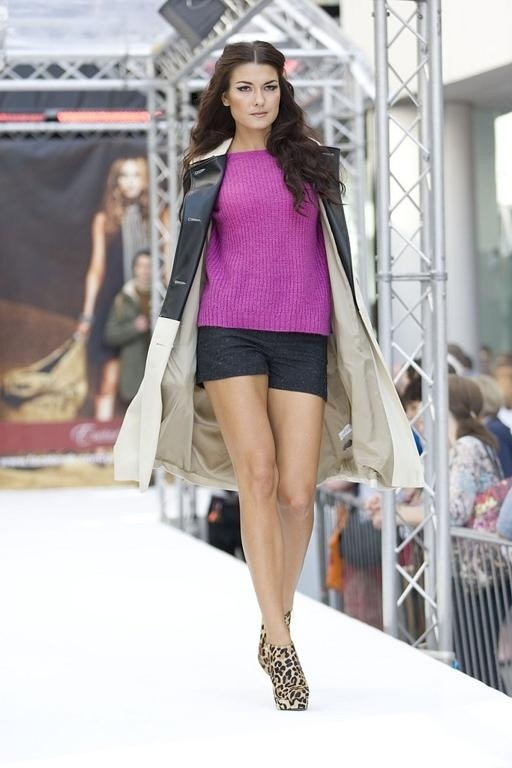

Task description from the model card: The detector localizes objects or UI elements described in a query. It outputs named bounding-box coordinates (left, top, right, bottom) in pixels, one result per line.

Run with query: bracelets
left=80, top=314, right=97, bottom=324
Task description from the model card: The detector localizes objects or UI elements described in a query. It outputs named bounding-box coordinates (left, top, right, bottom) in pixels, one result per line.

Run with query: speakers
left=159, top=0, right=226, bottom=48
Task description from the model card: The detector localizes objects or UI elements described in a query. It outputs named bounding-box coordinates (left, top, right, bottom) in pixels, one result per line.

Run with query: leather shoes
left=257, top=608, right=309, bottom=712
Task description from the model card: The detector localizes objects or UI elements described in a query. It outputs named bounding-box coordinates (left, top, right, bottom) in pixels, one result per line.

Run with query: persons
left=326, top=343, right=512, bottom=695
left=101, top=250, right=161, bottom=406
left=208, top=489, right=245, bottom=562
left=144, top=37, right=355, bottom=714
left=74, top=155, right=155, bottom=451
